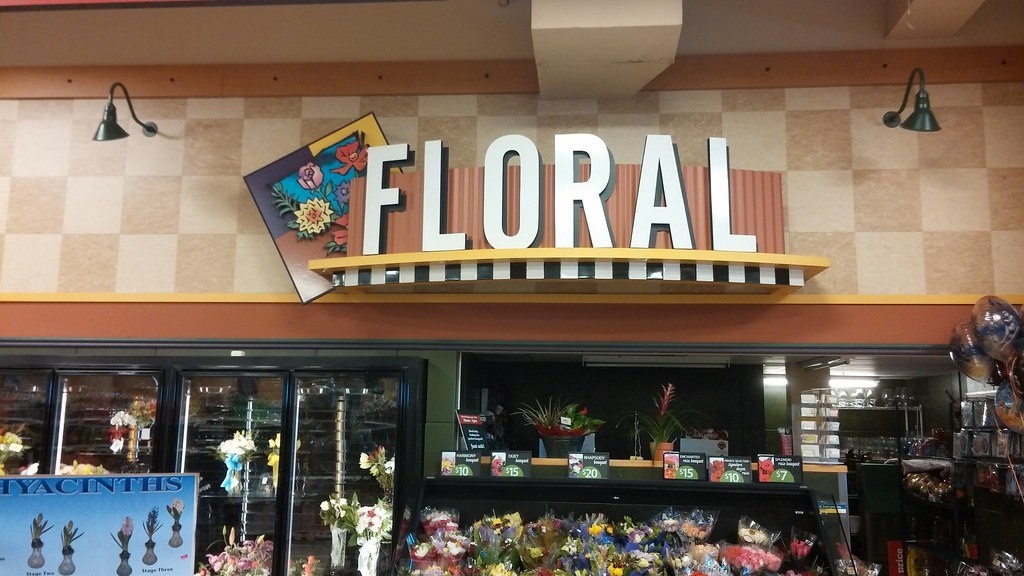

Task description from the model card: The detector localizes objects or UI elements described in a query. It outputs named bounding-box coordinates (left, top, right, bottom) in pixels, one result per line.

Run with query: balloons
left=948, top=295, right=1024, bottom=433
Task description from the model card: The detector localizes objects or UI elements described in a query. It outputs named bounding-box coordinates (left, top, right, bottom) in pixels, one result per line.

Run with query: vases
left=169, top=530, right=184, bottom=548
left=57, top=554, right=76, bottom=575
left=27, top=547, right=45, bottom=569
left=142, top=547, right=157, bottom=565
left=117, top=557, right=132, bottom=576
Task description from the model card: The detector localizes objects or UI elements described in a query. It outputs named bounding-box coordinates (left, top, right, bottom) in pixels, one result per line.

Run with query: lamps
left=91, top=82, right=157, bottom=141
left=882, top=68, right=941, bottom=131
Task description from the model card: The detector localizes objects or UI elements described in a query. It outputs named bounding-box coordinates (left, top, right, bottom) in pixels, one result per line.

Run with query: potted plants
left=509, top=394, right=607, bottom=458
left=615, top=383, right=719, bottom=466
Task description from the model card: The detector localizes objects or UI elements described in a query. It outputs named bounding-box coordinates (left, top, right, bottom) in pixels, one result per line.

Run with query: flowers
left=57, top=520, right=85, bottom=554
left=358, top=445, right=396, bottom=504
left=266, top=433, right=301, bottom=488
left=166, top=498, right=184, bottom=530
left=347, top=491, right=394, bottom=576
left=27, top=513, right=54, bottom=548
left=397, top=508, right=820, bottom=576
left=109, top=516, right=135, bottom=556
left=288, top=555, right=323, bottom=576
left=318, top=499, right=348, bottom=567
left=141, top=507, right=163, bottom=546
left=206, top=526, right=274, bottom=576
left=215, top=431, right=258, bottom=496
left=0, top=423, right=31, bottom=475
